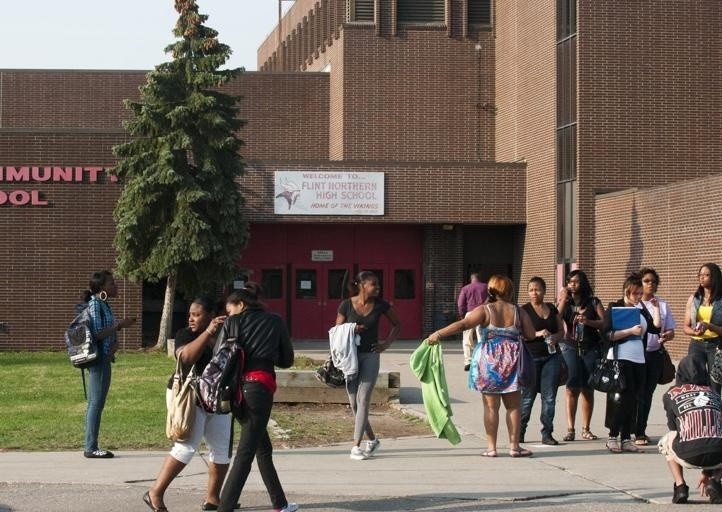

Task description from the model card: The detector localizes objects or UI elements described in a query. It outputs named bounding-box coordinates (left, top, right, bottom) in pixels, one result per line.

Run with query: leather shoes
left=464, top=363, right=470, bottom=370
left=203, top=499, right=241, bottom=510
left=143, top=490, right=168, bottom=512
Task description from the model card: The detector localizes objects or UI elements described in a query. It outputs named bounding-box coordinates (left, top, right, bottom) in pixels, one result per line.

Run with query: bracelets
left=116, top=322, right=122, bottom=331
left=436, top=331, right=442, bottom=338
left=206, top=328, right=215, bottom=337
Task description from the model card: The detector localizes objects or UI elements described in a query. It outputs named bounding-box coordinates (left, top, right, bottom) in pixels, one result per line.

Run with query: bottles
left=545, top=334, right=556, bottom=356
left=574, top=320, right=582, bottom=343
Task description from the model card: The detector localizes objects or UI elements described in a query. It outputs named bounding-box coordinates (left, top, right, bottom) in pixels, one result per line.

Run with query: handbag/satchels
left=657, top=346, right=676, bottom=384
left=313, top=354, right=346, bottom=388
left=164, top=350, right=197, bottom=442
left=587, top=329, right=627, bottom=393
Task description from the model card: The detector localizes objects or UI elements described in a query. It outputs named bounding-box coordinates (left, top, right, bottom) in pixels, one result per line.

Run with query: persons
left=557, top=270, right=608, bottom=440
left=213, top=282, right=300, bottom=511
left=519, top=277, right=565, bottom=445
left=458, top=271, right=488, bottom=371
left=331, top=270, right=402, bottom=462
left=143, top=296, right=232, bottom=510
left=426, top=275, right=536, bottom=456
left=606, top=275, right=661, bottom=453
left=633, top=268, right=677, bottom=446
left=84, top=269, right=138, bottom=457
left=684, top=263, right=722, bottom=394
left=657, top=354, right=722, bottom=504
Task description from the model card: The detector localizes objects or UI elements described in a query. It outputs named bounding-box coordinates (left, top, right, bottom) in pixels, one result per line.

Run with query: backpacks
left=195, top=317, right=245, bottom=414
left=64, top=299, right=104, bottom=400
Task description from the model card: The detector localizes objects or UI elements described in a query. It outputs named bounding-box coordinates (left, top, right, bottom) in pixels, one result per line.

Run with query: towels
left=411, top=338, right=463, bottom=448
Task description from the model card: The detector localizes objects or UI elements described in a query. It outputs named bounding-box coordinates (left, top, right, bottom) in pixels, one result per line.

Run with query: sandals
left=563, top=427, right=597, bottom=441
left=481, top=449, right=496, bottom=457
left=510, top=448, right=533, bottom=457
left=605, top=435, right=648, bottom=452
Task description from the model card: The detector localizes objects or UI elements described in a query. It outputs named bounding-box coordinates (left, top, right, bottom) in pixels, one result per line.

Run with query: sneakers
left=672, top=481, right=689, bottom=503
left=350, top=436, right=381, bottom=459
left=705, top=479, right=722, bottom=503
left=542, top=433, right=558, bottom=445
left=84, top=450, right=113, bottom=457
left=280, top=503, right=299, bottom=512
